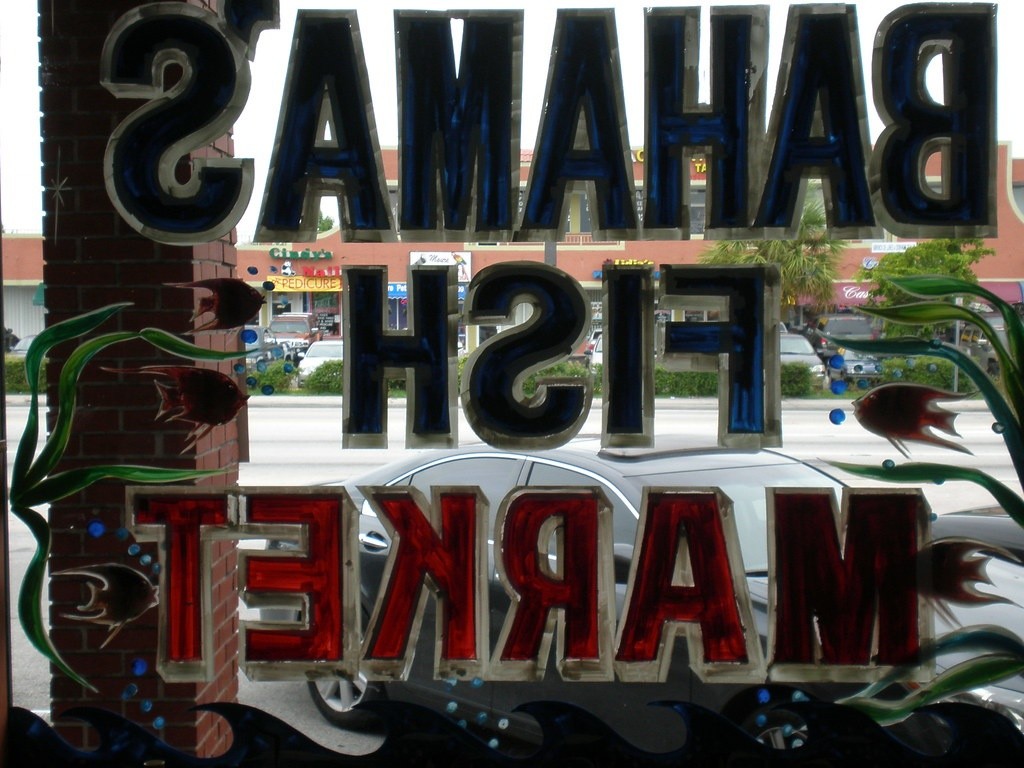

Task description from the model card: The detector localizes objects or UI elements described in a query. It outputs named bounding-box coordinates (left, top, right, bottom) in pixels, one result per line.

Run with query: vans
left=959, top=324, right=1012, bottom=375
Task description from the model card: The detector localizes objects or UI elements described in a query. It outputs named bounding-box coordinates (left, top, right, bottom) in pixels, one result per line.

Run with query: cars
left=587, top=328, right=603, bottom=377
left=296, top=335, right=343, bottom=388
left=780, top=313, right=886, bottom=391
left=8, top=335, right=45, bottom=358
left=268, top=312, right=323, bottom=364
left=254, top=433, right=914, bottom=768
left=244, top=325, right=277, bottom=370
left=906, top=505, right=1024, bottom=768
